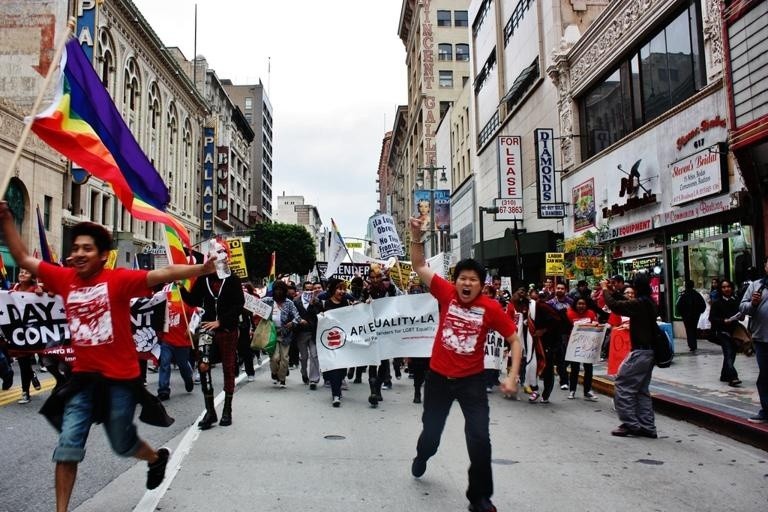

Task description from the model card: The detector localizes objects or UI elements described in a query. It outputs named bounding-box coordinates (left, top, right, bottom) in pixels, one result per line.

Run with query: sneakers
left=748, top=414, right=767, bottom=424
left=568, top=391, right=576, bottom=399
left=272, top=379, right=278, bottom=387
left=145, top=446, right=170, bottom=490
left=730, top=378, right=741, bottom=387
left=411, top=451, right=427, bottom=477
left=542, top=395, right=548, bottom=403
left=280, top=380, right=286, bottom=388
left=248, top=374, right=255, bottom=382
left=18, top=392, right=30, bottom=405
left=32, top=371, right=40, bottom=390
left=332, top=395, right=341, bottom=406
left=383, top=380, right=392, bottom=390
left=560, top=384, right=569, bottom=390
left=584, top=391, right=598, bottom=402
left=528, top=391, right=539, bottom=401
left=467, top=501, right=497, bottom=512
left=394, top=366, right=402, bottom=380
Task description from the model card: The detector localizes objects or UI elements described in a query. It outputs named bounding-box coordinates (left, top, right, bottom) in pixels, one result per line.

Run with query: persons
left=707, top=278, right=722, bottom=343
left=292, top=281, right=324, bottom=390
left=148, top=356, right=159, bottom=374
left=175, top=239, right=245, bottom=429
left=0, top=201, right=230, bottom=512
left=609, top=275, right=633, bottom=301
left=255, top=279, right=301, bottom=390
left=708, top=279, right=744, bottom=386
left=412, top=358, right=428, bottom=403
left=316, top=278, right=351, bottom=390
left=307, top=279, right=351, bottom=407
left=378, top=278, right=401, bottom=390
left=569, top=280, right=590, bottom=302
left=156, top=280, right=195, bottom=402
left=34, top=262, right=71, bottom=393
left=410, top=217, right=523, bottom=512
left=233, top=310, right=258, bottom=383
left=286, top=285, right=300, bottom=371
left=241, top=286, right=263, bottom=366
left=542, top=278, right=554, bottom=295
left=359, top=267, right=391, bottom=406
left=545, top=282, right=575, bottom=390
left=347, top=277, right=368, bottom=384
left=492, top=275, right=502, bottom=291
left=567, top=297, right=600, bottom=402
left=139, top=359, right=148, bottom=386
left=606, top=286, right=638, bottom=328
left=511, top=293, right=563, bottom=403
left=8, top=266, right=41, bottom=404
left=0, top=274, right=14, bottom=391
left=675, top=279, right=706, bottom=352
left=313, top=282, right=325, bottom=296
left=739, top=257, right=768, bottom=425
left=599, top=278, right=658, bottom=438
left=415, top=198, right=436, bottom=230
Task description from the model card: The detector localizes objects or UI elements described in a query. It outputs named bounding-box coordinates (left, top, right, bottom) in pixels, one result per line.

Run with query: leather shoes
left=155, top=392, right=170, bottom=401
left=613, top=424, right=641, bottom=436
left=639, top=428, right=657, bottom=436
left=185, top=376, right=194, bottom=393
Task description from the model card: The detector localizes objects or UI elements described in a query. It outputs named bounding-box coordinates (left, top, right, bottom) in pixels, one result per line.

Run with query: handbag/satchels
left=654, top=328, right=675, bottom=369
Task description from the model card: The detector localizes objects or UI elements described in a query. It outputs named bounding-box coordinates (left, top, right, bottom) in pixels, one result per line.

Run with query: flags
left=0, top=252, right=11, bottom=290
left=35, top=208, right=57, bottom=264
left=25, top=33, right=194, bottom=265
left=166, top=226, right=202, bottom=316
left=331, top=219, right=349, bottom=253
left=266, top=253, right=276, bottom=297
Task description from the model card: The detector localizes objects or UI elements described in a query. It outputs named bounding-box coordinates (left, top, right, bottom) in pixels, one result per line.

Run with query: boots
left=369, top=380, right=379, bottom=406
left=377, top=373, right=383, bottom=401
left=219, top=392, right=235, bottom=426
left=198, top=393, right=218, bottom=430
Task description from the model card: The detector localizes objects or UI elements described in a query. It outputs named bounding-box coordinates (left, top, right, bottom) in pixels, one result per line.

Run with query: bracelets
left=410, top=239, right=425, bottom=245
